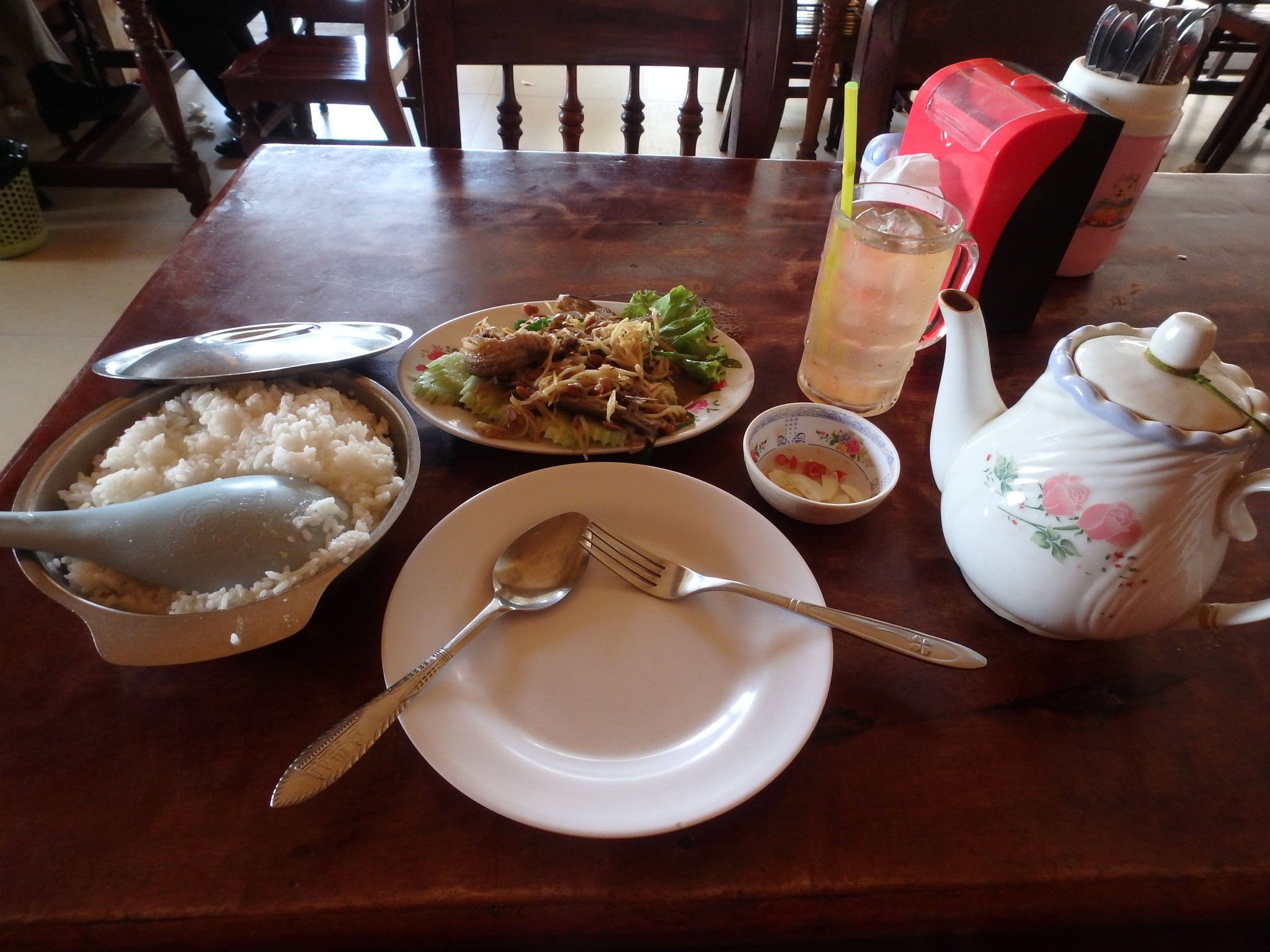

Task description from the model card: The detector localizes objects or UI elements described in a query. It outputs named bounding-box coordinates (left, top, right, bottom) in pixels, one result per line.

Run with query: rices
left=49, top=382, right=404, bottom=647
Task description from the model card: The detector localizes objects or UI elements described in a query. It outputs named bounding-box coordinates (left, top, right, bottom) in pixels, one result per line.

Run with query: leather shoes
left=35, top=79, right=144, bottom=134
left=224, top=109, right=243, bottom=124
left=214, top=121, right=292, bottom=158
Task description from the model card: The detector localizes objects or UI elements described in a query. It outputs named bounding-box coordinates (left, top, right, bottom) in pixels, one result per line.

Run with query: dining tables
left=7, top=140, right=1270, bottom=950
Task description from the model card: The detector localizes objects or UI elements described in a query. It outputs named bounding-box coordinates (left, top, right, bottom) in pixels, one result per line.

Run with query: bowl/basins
left=744, top=403, right=901, bottom=525
left=8, top=368, right=420, bottom=668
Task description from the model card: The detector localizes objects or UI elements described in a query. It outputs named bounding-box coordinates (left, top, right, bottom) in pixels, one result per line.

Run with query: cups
left=1055, top=53, right=1193, bottom=277
left=794, top=180, right=980, bottom=416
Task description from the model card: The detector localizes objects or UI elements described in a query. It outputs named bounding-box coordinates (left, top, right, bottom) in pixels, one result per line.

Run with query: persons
left=0, top=1, right=145, bottom=151
left=151, top=0, right=299, bottom=158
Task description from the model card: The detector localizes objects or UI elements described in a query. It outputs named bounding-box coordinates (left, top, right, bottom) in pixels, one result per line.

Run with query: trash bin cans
left=1, top=135, right=48, bottom=263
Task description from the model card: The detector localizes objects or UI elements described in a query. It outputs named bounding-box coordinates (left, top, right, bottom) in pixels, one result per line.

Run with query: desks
left=0, top=0, right=334, bottom=216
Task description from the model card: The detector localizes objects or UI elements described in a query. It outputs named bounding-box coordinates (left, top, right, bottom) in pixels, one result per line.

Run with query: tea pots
left=928, top=288, right=1270, bottom=643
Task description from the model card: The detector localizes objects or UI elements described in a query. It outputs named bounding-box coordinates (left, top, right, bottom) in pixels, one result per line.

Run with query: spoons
left=269, top=511, right=594, bottom=810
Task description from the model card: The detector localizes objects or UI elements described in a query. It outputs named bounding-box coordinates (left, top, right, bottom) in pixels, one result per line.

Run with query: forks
left=576, top=520, right=989, bottom=669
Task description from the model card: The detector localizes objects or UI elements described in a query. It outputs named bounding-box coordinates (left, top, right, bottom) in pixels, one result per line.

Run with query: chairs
left=710, top=0, right=1270, bottom=175
left=408, top=0, right=791, bottom=163
left=216, top=2, right=431, bottom=149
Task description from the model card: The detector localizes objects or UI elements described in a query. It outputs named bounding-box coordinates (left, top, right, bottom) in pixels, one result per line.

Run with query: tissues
left=865, top=54, right=1127, bottom=372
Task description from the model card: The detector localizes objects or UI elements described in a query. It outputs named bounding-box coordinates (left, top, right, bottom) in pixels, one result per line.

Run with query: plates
left=380, top=460, right=833, bottom=841
left=396, top=298, right=755, bottom=455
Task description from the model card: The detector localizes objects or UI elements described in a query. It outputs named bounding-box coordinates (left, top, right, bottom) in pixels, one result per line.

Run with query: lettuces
left=623, top=284, right=728, bottom=386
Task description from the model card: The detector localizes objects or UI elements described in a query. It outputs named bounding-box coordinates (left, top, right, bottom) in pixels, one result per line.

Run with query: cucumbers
left=411, top=351, right=627, bottom=450
